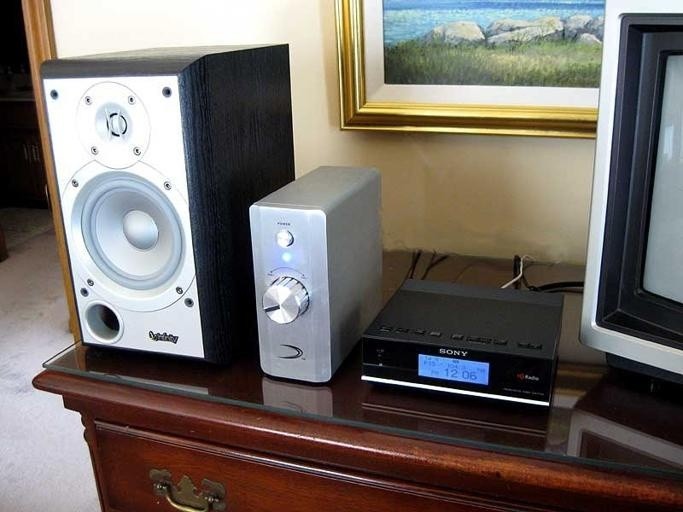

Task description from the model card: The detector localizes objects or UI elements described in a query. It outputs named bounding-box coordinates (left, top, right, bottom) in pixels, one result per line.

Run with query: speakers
left=39, top=43, right=296, bottom=369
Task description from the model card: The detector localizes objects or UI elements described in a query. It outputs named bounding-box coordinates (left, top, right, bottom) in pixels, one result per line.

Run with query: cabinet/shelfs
left=0, top=102, right=50, bottom=209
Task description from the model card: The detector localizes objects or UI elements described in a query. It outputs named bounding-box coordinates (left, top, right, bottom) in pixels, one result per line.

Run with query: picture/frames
left=335, top=0, right=608, bottom=140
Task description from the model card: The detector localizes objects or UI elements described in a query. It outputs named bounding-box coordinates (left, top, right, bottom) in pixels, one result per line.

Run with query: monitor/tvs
left=565, top=374, right=683, bottom=471
left=577, top=0, right=683, bottom=385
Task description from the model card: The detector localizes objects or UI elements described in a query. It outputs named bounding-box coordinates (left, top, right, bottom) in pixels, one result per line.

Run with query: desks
left=32, top=247, right=683, bottom=512
